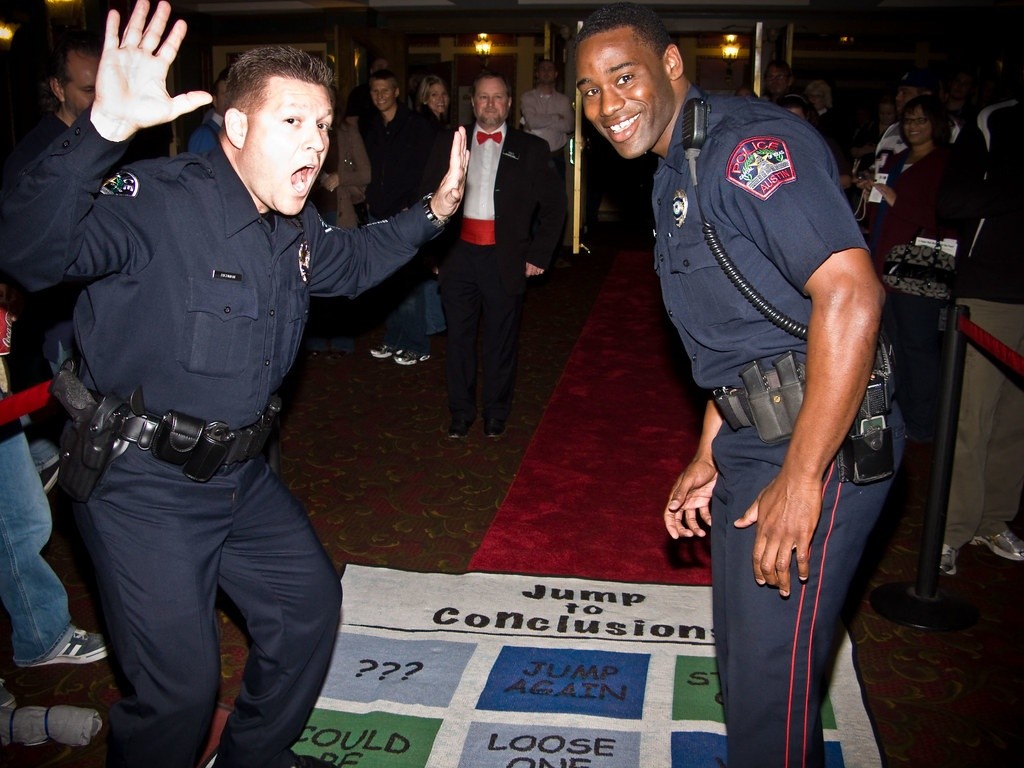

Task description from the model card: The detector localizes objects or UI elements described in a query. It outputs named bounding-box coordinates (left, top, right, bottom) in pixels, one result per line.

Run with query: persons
left=402, top=63, right=566, bottom=437
left=575, top=0, right=905, bottom=768
left=0, top=0, right=456, bottom=710
left=521, top=59, right=575, bottom=254
left=735, top=60, right=1024, bottom=573
left=0, top=0, right=472, bottom=767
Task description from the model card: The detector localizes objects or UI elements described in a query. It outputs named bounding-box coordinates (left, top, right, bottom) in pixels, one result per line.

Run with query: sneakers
left=16, top=629, right=113, bottom=668
left=368, top=343, right=397, bottom=359
left=937, top=544, right=959, bottom=576
left=0, top=679, right=18, bottom=709
left=967, top=529, right=1024, bottom=560
left=393, top=347, right=431, bottom=365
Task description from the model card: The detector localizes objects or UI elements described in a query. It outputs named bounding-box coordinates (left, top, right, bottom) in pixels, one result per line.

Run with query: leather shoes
left=448, top=420, right=474, bottom=438
left=484, top=416, right=507, bottom=438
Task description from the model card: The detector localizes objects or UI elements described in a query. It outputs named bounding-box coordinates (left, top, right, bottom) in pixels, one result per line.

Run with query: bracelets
left=422, top=191, right=451, bottom=228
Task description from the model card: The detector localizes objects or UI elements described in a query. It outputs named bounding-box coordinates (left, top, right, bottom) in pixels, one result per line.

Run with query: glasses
left=902, top=117, right=928, bottom=125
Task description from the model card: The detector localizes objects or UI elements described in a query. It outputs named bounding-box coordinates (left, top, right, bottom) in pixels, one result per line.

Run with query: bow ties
left=477, top=131, right=502, bottom=145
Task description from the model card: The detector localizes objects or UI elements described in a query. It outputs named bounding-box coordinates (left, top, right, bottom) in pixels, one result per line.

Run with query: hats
left=896, top=69, right=934, bottom=88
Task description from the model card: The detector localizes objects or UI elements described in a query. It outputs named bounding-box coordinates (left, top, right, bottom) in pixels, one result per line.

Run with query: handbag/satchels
left=881, top=199, right=956, bottom=300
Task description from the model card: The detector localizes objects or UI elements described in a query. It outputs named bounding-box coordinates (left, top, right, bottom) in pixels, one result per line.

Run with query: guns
left=48, top=366, right=131, bottom=504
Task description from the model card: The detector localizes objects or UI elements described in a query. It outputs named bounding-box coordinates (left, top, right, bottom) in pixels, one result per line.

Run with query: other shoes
left=288, top=756, right=337, bottom=768
left=40, top=461, right=60, bottom=494
